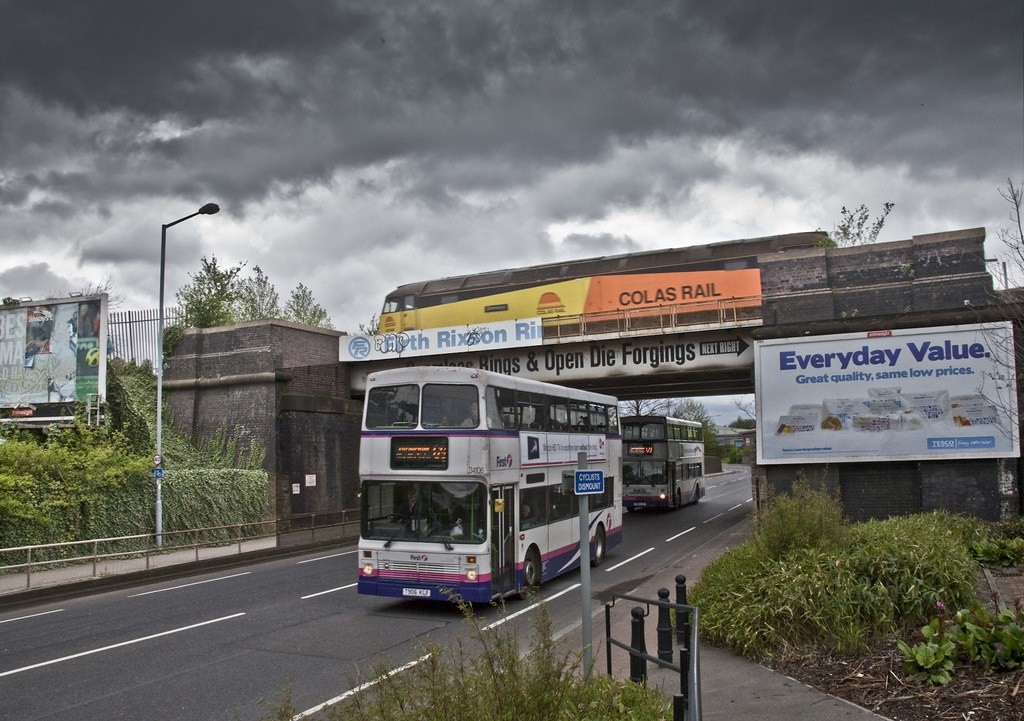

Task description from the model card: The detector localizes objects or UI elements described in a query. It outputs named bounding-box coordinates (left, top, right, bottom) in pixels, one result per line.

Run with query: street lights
left=155, top=203, right=221, bottom=551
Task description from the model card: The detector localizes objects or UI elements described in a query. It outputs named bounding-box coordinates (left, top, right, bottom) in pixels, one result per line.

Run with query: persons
left=448, top=496, right=472, bottom=536
left=520, top=504, right=531, bottom=529
left=462, top=400, right=492, bottom=429
left=390, top=487, right=429, bottom=522
left=583, top=417, right=594, bottom=432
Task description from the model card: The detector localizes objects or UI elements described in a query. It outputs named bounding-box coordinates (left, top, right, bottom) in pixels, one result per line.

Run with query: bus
left=620, top=414, right=705, bottom=513
left=356, top=366, right=625, bottom=606
